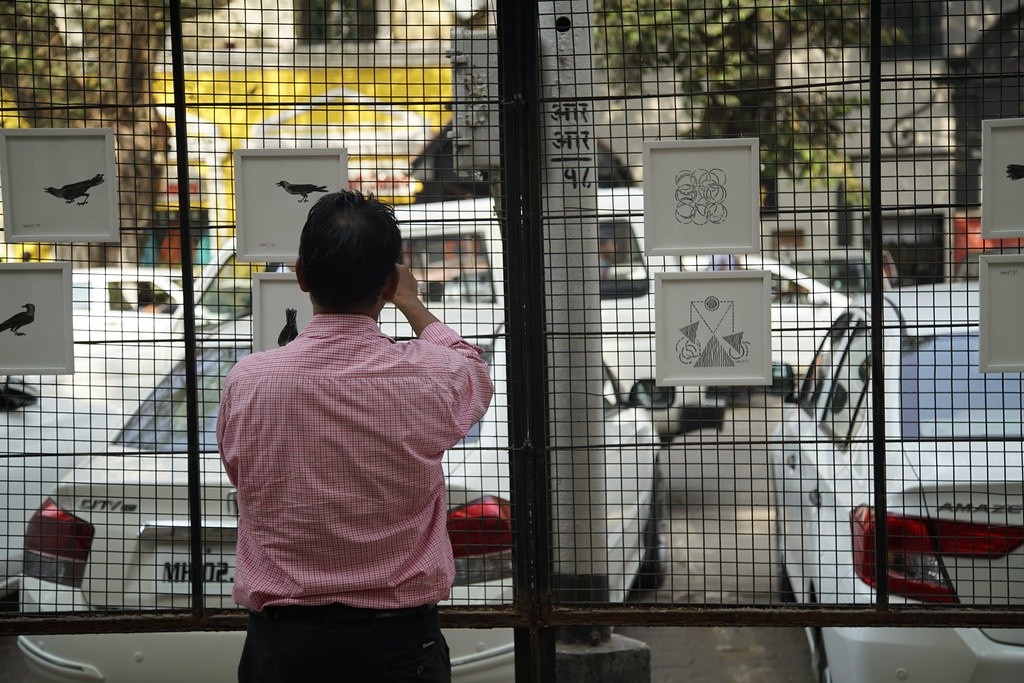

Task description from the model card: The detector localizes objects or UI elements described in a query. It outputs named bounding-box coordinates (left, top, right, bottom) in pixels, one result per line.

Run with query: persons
left=216, top=189, right=494, bottom=683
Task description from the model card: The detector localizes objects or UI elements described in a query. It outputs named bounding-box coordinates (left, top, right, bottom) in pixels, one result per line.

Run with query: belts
left=278, top=604, right=438, bottom=620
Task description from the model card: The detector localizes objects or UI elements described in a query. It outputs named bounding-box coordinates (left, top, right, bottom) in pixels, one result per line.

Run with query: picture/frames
left=252, top=273, right=313, bottom=352
left=3, top=127, right=122, bottom=244
left=234, top=148, right=350, bottom=262
left=981, top=116, right=1024, bottom=239
left=980, top=253, right=1024, bottom=372
left=643, top=136, right=762, bottom=257
left=655, top=269, right=774, bottom=387
left=0, top=259, right=75, bottom=375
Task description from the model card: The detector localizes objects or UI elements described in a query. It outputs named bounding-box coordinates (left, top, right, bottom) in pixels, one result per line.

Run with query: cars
left=16, top=312, right=683, bottom=683
left=755, top=283, right=1024, bottom=683
left=2, top=185, right=908, bottom=606
